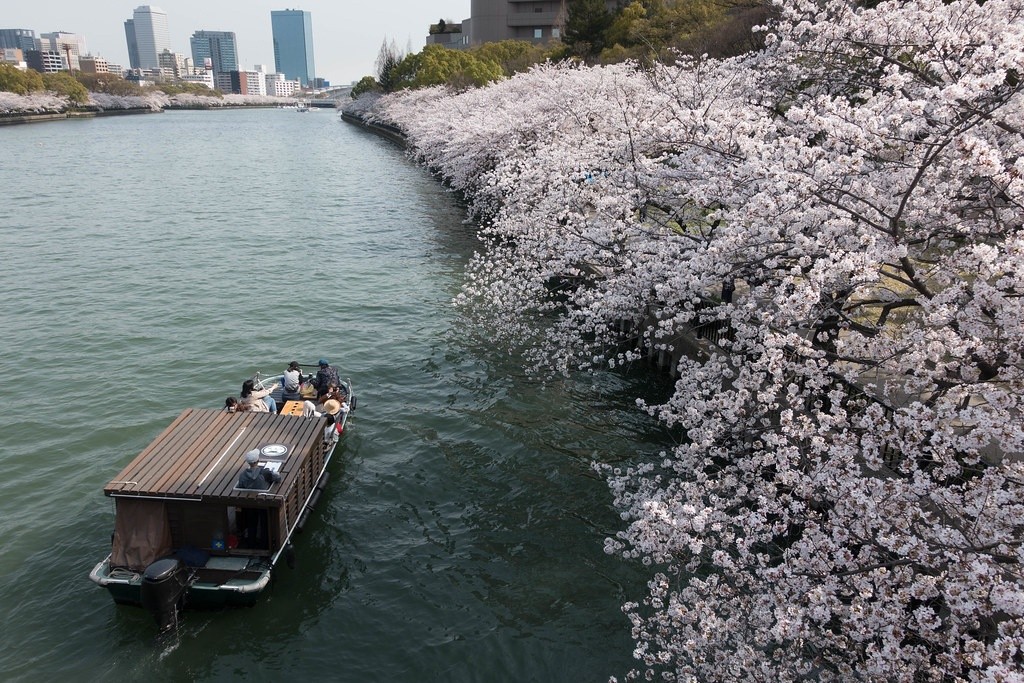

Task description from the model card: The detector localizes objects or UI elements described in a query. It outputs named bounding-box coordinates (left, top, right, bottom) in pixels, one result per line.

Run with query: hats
left=245, top=449, right=260, bottom=463
left=290, top=361, right=298, bottom=366
left=324, top=399, right=341, bottom=414
left=319, top=360, right=328, bottom=365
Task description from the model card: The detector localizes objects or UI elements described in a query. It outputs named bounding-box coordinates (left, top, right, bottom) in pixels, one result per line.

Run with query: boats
left=88, top=371, right=357, bottom=616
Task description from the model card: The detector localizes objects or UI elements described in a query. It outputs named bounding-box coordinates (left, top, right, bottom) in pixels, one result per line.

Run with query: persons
left=226, top=397, right=237, bottom=412
left=239, top=449, right=281, bottom=549
left=240, top=380, right=281, bottom=414
left=321, top=398, right=341, bottom=444
left=311, top=359, right=341, bottom=392
left=284, top=361, right=303, bottom=393
